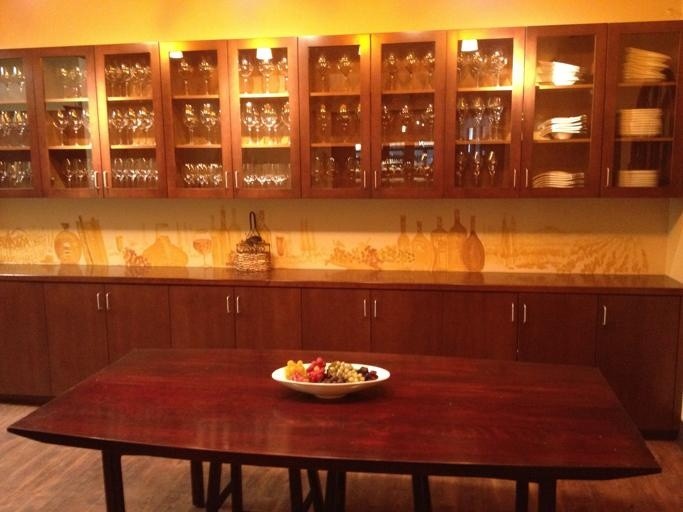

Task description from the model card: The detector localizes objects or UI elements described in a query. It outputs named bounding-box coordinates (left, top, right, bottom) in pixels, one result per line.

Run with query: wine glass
left=52, top=55, right=94, bottom=190
left=0, top=56, right=33, bottom=190
left=455, top=40, right=515, bottom=190
left=310, top=46, right=361, bottom=187
left=105, top=54, right=161, bottom=191
left=169, top=51, right=225, bottom=189
left=238, top=50, right=292, bottom=190
left=382, top=43, right=435, bottom=187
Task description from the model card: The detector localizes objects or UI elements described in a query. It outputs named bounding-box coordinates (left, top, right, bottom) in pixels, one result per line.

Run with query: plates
left=617, top=45, right=672, bottom=188
left=271, top=362, right=391, bottom=400
left=531, top=59, right=592, bottom=188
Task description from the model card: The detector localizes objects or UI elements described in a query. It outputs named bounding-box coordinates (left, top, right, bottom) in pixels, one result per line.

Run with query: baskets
left=236, top=242, right=271, bottom=273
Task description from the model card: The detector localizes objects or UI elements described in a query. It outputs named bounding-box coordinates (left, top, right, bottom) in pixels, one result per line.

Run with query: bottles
left=54, top=222, right=82, bottom=266
left=397, top=208, right=485, bottom=272
left=256, top=209, right=273, bottom=255
left=208, top=207, right=243, bottom=269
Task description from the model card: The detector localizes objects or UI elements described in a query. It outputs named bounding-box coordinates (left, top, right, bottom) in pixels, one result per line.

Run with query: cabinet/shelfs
left=299, top=30, right=447, bottom=198
left=1, top=263, right=682, bottom=437
left=2, top=47, right=41, bottom=198
left=43, top=42, right=168, bottom=198
left=168, top=36, right=299, bottom=198
left=600, top=19, right=683, bottom=197
left=443, top=20, right=609, bottom=198
